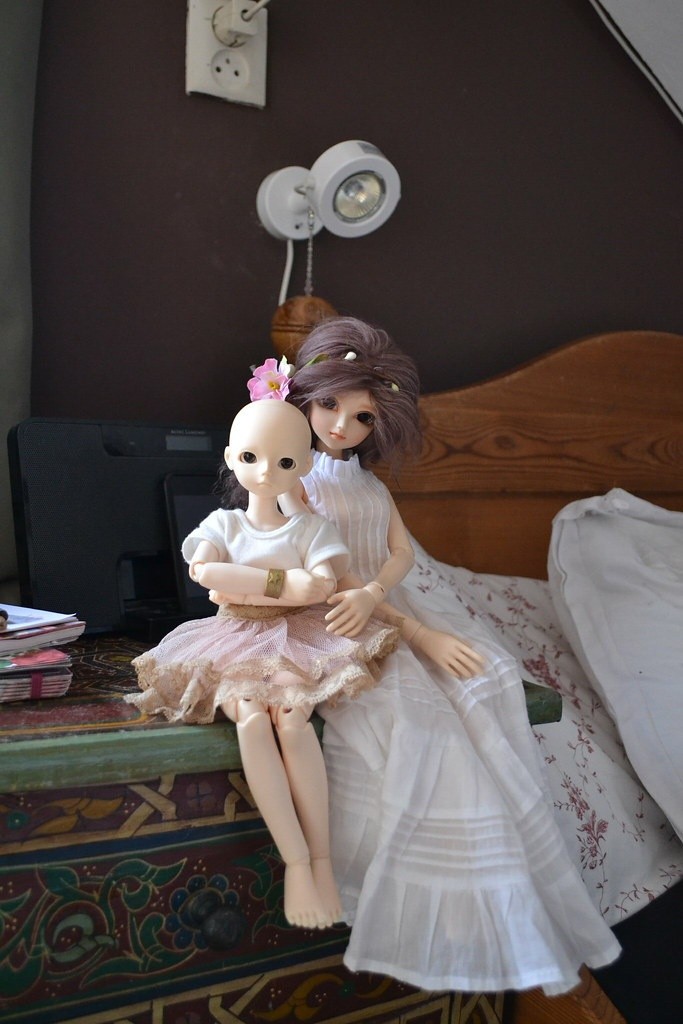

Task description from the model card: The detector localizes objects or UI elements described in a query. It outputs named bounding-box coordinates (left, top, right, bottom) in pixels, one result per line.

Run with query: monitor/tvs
left=8, top=418, right=250, bottom=651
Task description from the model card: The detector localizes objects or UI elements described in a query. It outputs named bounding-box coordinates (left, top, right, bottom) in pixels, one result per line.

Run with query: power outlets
left=187, top=0, right=267, bottom=109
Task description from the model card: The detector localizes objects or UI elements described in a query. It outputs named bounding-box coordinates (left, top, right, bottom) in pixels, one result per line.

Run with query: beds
left=367, top=331, right=683, bottom=1024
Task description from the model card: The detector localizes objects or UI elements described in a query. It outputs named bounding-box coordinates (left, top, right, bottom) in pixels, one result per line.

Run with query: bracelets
left=264, top=568, right=285, bottom=599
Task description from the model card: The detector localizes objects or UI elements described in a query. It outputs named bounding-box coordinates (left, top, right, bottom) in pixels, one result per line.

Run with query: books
left=0, top=603, right=85, bottom=702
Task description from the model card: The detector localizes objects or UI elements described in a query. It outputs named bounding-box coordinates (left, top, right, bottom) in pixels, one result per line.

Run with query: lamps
left=255, top=139, right=401, bottom=306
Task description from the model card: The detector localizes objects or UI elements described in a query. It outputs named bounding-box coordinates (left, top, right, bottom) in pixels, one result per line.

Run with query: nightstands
left=0, top=638, right=564, bottom=1024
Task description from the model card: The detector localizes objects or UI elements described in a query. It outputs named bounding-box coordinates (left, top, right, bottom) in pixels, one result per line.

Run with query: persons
left=123, top=397, right=400, bottom=929
left=225, top=319, right=623, bottom=994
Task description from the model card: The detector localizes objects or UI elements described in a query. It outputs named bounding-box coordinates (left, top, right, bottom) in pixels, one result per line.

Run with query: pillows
left=548, top=489, right=683, bottom=843
left=399, top=523, right=683, bottom=927
